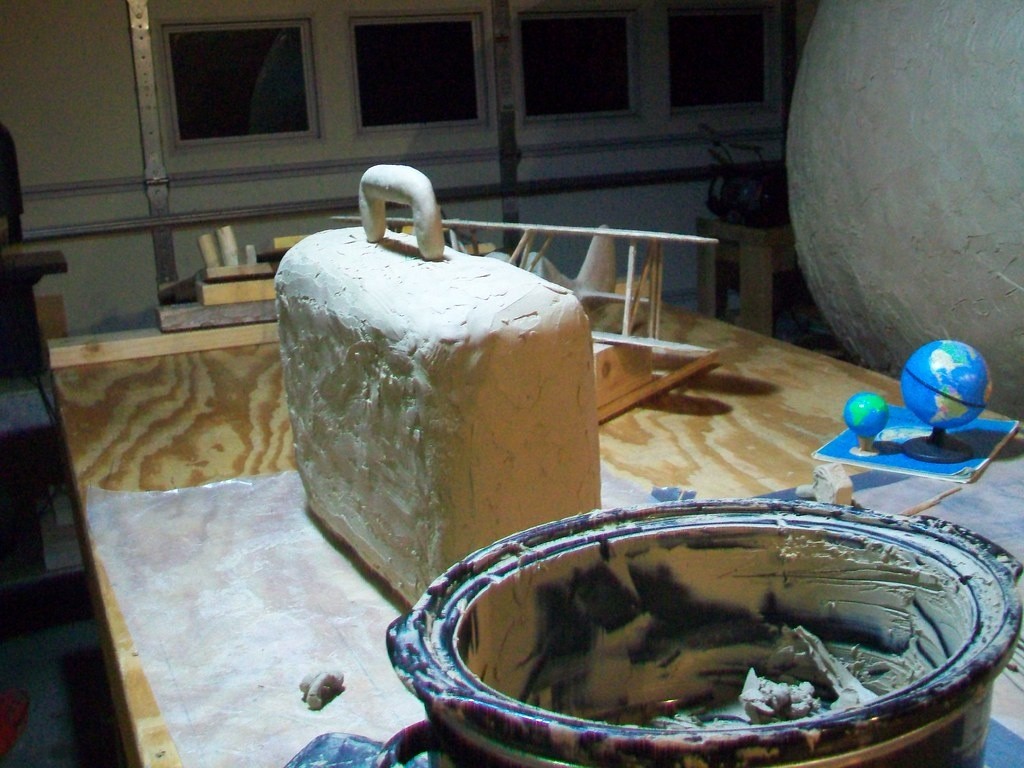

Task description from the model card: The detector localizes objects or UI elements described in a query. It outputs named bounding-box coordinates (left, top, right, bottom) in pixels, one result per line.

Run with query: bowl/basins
left=386, top=500, right=1024, bottom=768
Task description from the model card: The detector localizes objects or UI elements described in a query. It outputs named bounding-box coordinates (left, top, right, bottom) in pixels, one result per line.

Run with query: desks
left=696, top=220, right=796, bottom=337
left=50, top=280, right=1024, bottom=768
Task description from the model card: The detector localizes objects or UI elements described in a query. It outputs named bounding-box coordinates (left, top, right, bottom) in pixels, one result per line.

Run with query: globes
left=897, top=340, right=994, bottom=464
left=842, top=391, right=890, bottom=457
